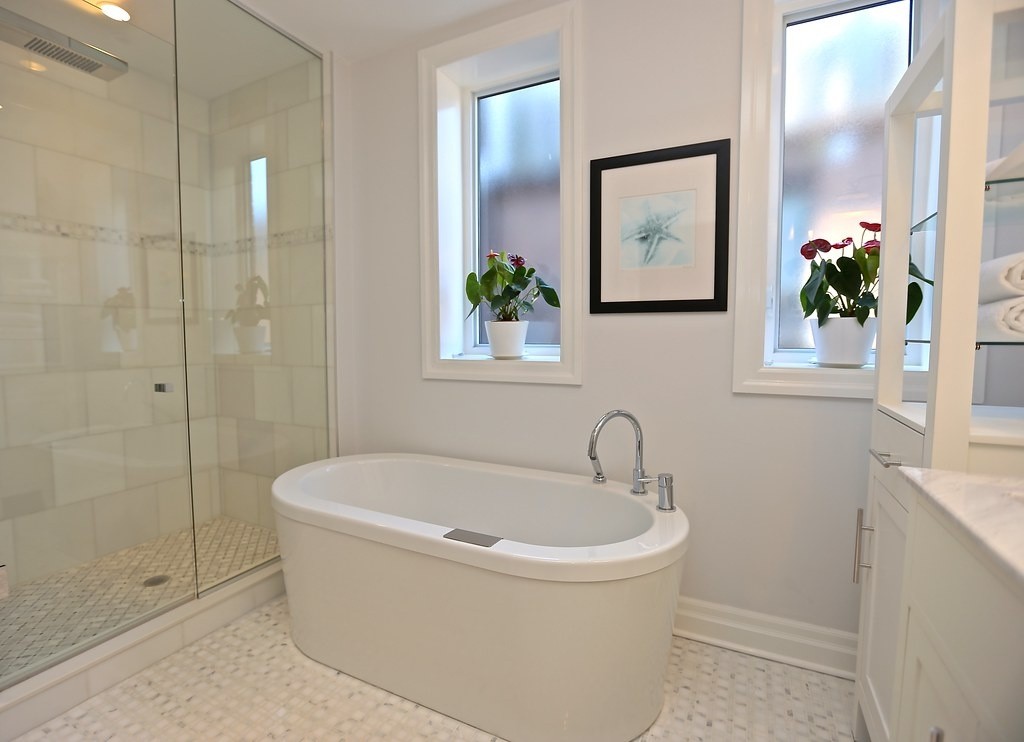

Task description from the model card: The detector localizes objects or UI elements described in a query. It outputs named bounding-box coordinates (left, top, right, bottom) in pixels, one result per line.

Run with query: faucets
left=589, top=409, right=644, bottom=495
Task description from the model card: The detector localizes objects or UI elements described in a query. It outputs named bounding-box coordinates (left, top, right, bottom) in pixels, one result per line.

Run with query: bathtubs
left=272, top=452, right=691, bottom=741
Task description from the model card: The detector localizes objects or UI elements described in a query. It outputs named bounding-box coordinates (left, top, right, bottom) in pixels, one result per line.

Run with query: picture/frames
left=589, top=137, right=730, bottom=313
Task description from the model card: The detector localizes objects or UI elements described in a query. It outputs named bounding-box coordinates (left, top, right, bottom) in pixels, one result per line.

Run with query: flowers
left=465, top=250, right=560, bottom=322
left=800, top=222, right=934, bottom=328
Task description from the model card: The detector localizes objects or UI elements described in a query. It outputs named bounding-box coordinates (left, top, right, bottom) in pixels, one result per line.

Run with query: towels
left=985, top=144, right=1024, bottom=208
left=977, top=246, right=1023, bottom=345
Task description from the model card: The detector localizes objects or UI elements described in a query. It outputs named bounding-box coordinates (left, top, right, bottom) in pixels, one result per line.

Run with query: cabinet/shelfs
left=851, top=0, right=1024, bottom=742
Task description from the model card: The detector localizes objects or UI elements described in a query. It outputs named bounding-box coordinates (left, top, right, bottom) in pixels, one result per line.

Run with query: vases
left=485, top=321, right=529, bottom=359
left=808, top=317, right=877, bottom=368
